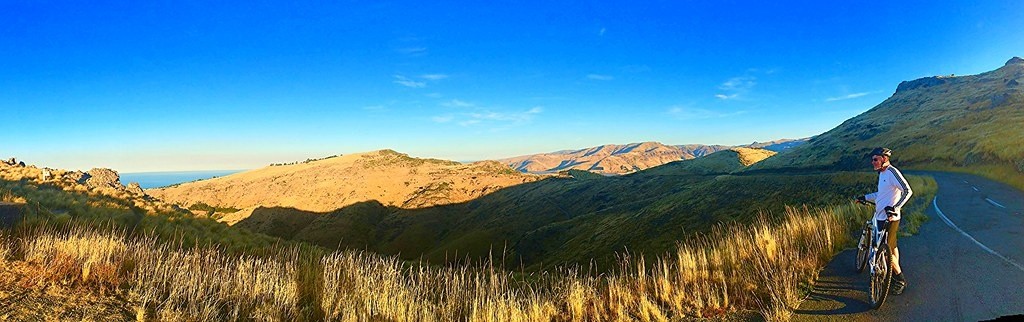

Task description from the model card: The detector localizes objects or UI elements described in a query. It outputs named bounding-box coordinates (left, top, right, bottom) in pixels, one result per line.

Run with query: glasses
left=870, top=157, right=878, bottom=161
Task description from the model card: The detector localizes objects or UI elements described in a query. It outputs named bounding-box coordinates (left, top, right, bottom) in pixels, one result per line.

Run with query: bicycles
left=854, top=195, right=898, bottom=310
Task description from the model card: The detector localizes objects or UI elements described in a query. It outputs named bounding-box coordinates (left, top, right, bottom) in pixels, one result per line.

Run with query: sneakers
left=892, top=279, right=907, bottom=295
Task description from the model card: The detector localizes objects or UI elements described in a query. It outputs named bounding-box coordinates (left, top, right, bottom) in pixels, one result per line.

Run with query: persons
left=855, top=147, right=914, bottom=296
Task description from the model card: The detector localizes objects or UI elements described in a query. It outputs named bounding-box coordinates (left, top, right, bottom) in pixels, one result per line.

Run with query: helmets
left=865, top=147, right=893, bottom=158
